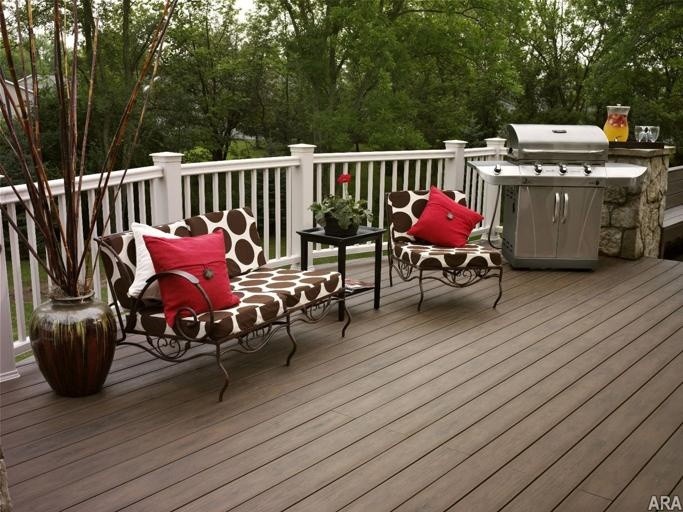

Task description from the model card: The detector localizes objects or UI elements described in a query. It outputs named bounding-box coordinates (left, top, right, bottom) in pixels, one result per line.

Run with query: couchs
left=94, top=207, right=352, bottom=403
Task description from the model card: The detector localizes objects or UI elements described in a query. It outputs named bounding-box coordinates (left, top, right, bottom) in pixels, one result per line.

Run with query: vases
left=324, top=215, right=359, bottom=237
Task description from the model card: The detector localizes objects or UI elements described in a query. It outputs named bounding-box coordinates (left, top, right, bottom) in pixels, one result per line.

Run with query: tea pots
left=603, top=103, right=631, bottom=142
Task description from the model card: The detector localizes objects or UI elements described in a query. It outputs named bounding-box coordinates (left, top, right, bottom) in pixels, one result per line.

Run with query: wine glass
left=635, top=126, right=646, bottom=142
left=648, top=126, right=661, bottom=143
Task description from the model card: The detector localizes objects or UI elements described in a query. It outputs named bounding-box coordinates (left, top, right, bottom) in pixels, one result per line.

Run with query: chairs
left=383, top=190, right=504, bottom=312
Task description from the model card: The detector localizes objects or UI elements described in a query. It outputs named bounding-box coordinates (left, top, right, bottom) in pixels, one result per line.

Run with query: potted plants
left=0, top=1, right=179, bottom=396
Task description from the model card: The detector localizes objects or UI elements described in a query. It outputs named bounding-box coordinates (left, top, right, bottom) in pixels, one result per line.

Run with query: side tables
left=297, top=226, right=388, bottom=322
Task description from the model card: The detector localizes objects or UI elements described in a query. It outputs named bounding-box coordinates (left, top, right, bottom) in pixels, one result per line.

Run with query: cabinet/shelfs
left=503, top=186, right=603, bottom=271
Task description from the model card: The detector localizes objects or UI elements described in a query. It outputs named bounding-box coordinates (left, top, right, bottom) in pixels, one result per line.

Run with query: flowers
left=308, top=175, right=374, bottom=229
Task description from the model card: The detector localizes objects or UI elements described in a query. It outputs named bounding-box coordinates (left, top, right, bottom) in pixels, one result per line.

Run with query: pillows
left=127, top=222, right=183, bottom=301
left=142, top=228, right=239, bottom=329
left=407, top=185, right=484, bottom=247
